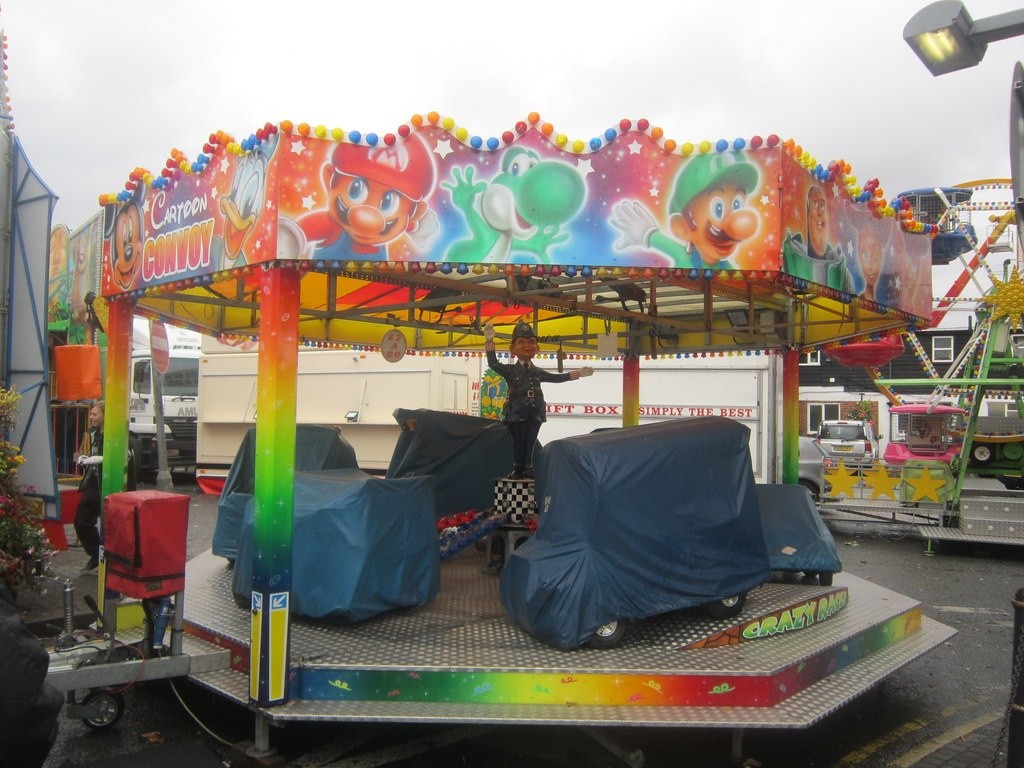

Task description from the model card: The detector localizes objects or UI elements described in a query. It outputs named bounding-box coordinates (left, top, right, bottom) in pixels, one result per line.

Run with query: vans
left=816, top=419, right=884, bottom=468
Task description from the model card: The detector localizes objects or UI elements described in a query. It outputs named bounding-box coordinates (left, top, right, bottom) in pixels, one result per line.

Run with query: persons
left=484, top=322, right=594, bottom=479
left=74, top=401, right=134, bottom=574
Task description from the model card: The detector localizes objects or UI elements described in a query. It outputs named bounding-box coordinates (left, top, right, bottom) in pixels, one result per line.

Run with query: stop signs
left=150, top=316, right=170, bottom=374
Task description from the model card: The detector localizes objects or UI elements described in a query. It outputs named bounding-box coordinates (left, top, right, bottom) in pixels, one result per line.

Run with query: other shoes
left=86, top=554, right=99, bottom=570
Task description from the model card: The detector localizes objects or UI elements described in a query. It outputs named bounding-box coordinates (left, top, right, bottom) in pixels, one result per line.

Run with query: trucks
left=77, top=291, right=201, bottom=482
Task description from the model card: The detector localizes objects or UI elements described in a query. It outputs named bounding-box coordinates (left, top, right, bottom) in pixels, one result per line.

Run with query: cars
left=798, top=437, right=834, bottom=504
